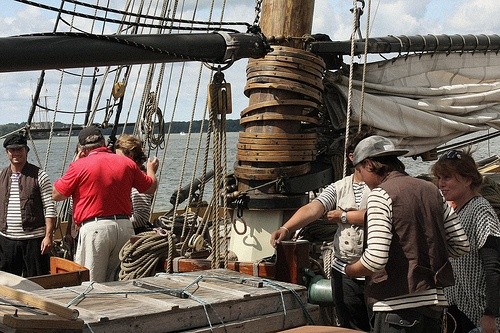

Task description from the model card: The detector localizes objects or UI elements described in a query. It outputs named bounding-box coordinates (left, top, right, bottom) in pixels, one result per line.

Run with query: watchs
left=341, top=211, right=346, bottom=225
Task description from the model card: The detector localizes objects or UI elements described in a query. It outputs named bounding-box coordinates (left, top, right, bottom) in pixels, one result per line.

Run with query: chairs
left=24, top=257, right=91, bottom=290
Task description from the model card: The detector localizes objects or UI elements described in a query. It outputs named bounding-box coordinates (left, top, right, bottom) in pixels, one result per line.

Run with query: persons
left=114, top=135, right=152, bottom=234
left=431, top=150, right=500, bottom=333
left=345, top=135, right=470, bottom=333
left=270, top=135, right=372, bottom=333
left=0, top=133, right=56, bottom=278
left=52, top=126, right=159, bottom=283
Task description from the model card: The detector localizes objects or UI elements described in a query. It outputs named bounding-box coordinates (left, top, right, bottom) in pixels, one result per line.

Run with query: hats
left=352, top=135, right=410, bottom=166
left=3, top=134, right=28, bottom=149
left=79, top=126, right=103, bottom=147
left=114, top=135, right=142, bottom=150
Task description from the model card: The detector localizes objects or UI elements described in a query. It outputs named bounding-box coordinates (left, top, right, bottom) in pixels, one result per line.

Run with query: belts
left=80, top=214, right=130, bottom=227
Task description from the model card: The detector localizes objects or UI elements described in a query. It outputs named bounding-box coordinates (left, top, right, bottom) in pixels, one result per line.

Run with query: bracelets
left=345, top=264, right=351, bottom=275
left=279, top=226, right=290, bottom=235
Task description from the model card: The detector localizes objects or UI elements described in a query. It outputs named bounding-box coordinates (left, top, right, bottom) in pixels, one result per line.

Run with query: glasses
left=441, top=151, right=462, bottom=161
